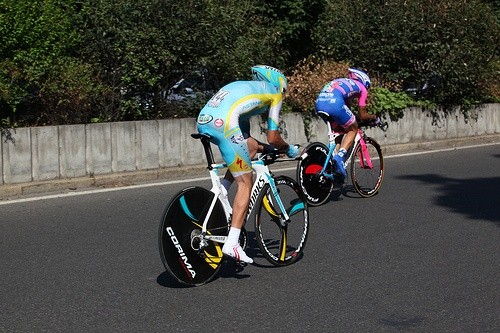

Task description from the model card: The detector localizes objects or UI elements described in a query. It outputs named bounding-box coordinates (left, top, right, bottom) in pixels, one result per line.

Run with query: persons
left=197, top=64, right=299, bottom=263
left=315, top=67, right=381, bottom=176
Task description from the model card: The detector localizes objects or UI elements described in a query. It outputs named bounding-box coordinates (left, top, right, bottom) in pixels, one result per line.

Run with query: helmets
left=251, top=65, right=288, bottom=94
left=347, top=68, right=370, bottom=90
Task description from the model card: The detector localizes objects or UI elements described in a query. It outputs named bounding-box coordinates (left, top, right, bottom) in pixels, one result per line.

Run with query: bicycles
left=295, top=110, right=384, bottom=207
left=157, top=133, right=309, bottom=287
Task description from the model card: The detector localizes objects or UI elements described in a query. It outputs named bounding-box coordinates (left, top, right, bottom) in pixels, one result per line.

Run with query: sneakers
left=222, top=243, right=254, bottom=263
left=332, top=155, right=347, bottom=178
left=209, top=183, right=233, bottom=214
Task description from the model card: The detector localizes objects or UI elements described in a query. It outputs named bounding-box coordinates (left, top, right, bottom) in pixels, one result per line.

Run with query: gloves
left=286, top=144, right=298, bottom=157
left=372, top=114, right=381, bottom=125
